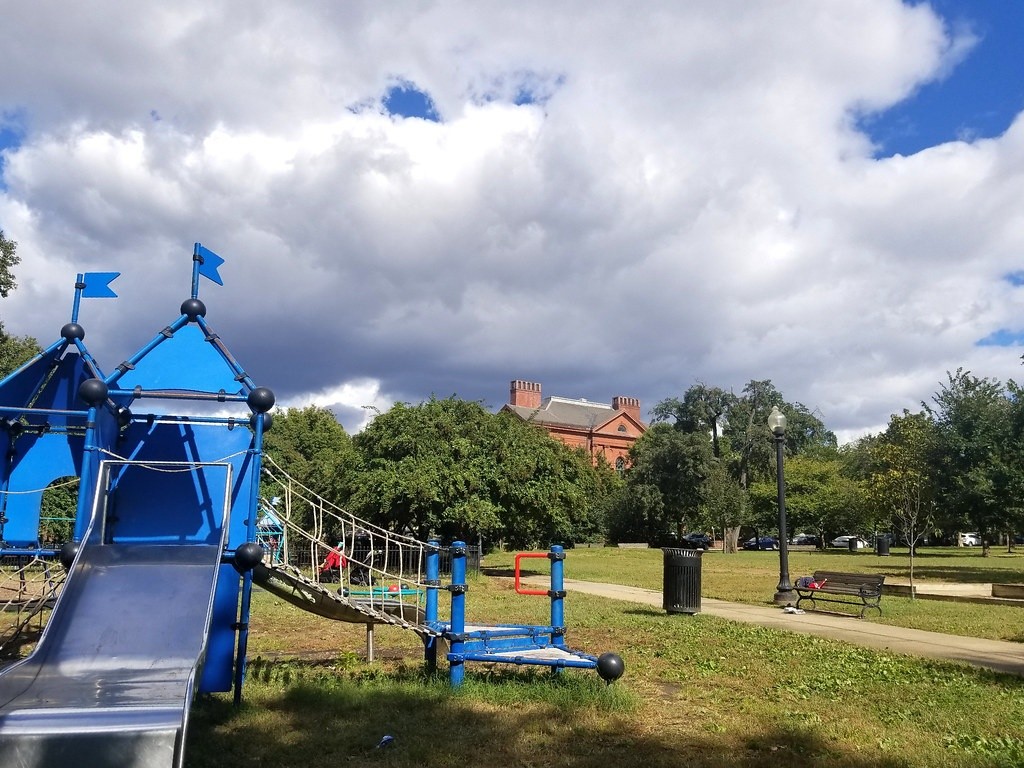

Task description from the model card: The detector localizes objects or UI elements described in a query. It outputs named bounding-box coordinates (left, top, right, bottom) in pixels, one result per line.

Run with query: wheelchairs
left=315, top=562, right=344, bottom=585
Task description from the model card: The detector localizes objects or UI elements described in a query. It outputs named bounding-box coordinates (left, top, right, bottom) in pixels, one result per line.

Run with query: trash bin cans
left=876, top=533, right=890, bottom=556
left=848, top=537, right=858, bottom=552
left=660, top=547, right=705, bottom=615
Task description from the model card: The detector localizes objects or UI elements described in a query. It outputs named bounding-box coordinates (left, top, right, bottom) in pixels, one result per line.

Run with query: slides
left=0, top=541, right=224, bottom=768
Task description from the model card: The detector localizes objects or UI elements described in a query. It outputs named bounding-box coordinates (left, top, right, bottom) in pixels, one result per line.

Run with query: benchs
left=792, top=570, right=885, bottom=618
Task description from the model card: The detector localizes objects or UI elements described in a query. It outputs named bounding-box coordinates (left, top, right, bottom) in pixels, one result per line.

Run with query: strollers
left=344, top=549, right=383, bottom=587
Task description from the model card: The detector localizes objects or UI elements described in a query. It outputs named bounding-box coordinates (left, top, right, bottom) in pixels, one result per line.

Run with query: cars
left=924, top=531, right=997, bottom=547
left=787, top=532, right=818, bottom=545
left=682, top=531, right=715, bottom=550
left=743, top=536, right=779, bottom=551
left=826, top=535, right=869, bottom=548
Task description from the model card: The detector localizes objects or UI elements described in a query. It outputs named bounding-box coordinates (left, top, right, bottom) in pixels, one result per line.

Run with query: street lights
left=767, top=407, right=798, bottom=601
